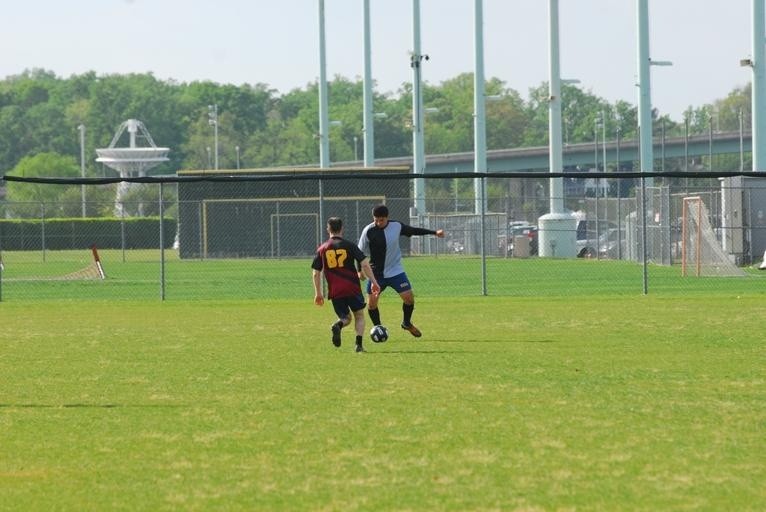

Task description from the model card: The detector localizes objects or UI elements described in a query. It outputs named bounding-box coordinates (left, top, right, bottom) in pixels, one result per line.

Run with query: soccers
left=369, top=325, right=388, bottom=342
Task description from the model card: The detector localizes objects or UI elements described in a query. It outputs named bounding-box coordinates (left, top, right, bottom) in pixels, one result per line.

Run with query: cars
left=412, top=222, right=538, bottom=256
left=577, top=215, right=721, bottom=258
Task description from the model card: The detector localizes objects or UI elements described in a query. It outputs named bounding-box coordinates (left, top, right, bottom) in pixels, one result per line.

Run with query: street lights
left=77, top=124, right=86, bottom=217
left=206, top=104, right=240, bottom=170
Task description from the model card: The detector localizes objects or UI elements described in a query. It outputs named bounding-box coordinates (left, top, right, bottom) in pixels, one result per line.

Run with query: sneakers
left=356, top=345, right=367, bottom=352
left=401, top=322, right=422, bottom=337
left=331, top=324, right=341, bottom=347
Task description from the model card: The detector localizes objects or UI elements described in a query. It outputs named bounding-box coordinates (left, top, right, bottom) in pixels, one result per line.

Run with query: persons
left=355, top=203, right=445, bottom=338
left=312, top=216, right=381, bottom=352
